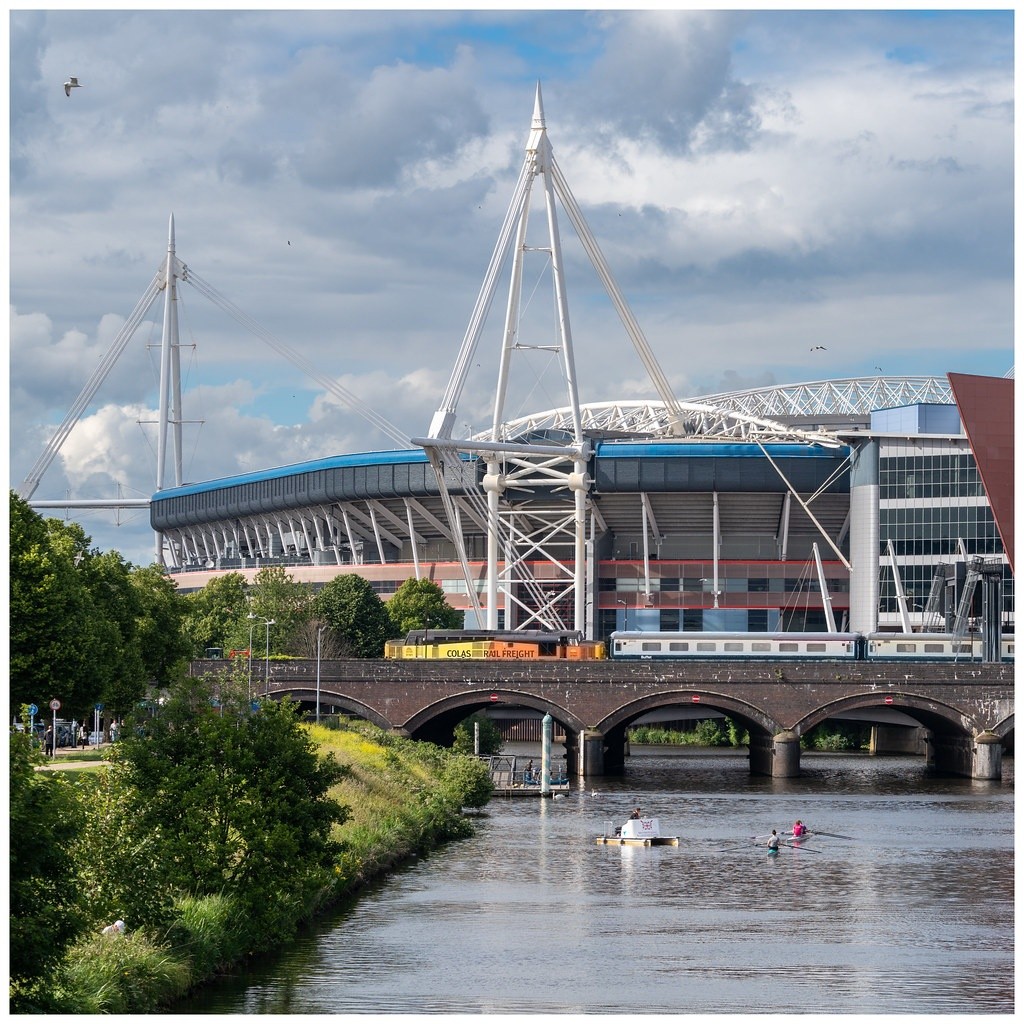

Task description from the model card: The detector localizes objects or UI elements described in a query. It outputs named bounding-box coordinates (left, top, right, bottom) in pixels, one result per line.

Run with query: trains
left=383, top=631, right=1014, bottom=662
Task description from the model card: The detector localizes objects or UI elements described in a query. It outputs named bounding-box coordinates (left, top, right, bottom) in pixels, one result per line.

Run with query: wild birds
left=875, top=366, right=881, bottom=371
left=64, top=77, right=83, bottom=97
left=810, top=346, right=826, bottom=351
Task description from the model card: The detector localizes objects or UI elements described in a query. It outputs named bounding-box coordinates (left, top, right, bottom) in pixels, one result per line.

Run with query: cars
left=228, top=649, right=249, bottom=658
left=13, top=722, right=104, bottom=748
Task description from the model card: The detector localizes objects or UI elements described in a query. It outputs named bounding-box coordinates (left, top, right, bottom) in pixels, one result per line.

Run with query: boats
left=591, top=833, right=679, bottom=849
left=787, top=825, right=814, bottom=844
left=450, top=755, right=569, bottom=797
left=765, top=848, right=780, bottom=856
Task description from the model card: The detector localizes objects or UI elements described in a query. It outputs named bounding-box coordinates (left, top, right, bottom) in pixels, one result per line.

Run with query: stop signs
left=692, top=695, right=700, bottom=703
left=885, top=696, right=893, bottom=705
left=489, top=692, right=500, bottom=703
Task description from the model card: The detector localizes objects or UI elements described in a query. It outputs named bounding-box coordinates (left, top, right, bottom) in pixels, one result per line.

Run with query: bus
left=205, top=647, right=223, bottom=659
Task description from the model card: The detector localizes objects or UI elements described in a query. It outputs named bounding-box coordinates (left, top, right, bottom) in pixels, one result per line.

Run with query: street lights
left=248, top=619, right=276, bottom=716
left=247, top=614, right=269, bottom=712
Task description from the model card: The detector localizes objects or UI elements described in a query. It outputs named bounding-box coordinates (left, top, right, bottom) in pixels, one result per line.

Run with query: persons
left=57, top=723, right=87, bottom=749
left=524, top=760, right=533, bottom=780
left=110, top=718, right=149, bottom=743
left=767, top=830, right=780, bottom=854
left=630, top=808, right=641, bottom=819
left=793, top=820, right=809, bottom=837
left=44, top=726, right=54, bottom=756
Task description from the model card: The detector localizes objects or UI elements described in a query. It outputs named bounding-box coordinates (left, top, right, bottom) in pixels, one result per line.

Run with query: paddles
left=777, top=843, right=822, bottom=853
left=809, top=829, right=853, bottom=840
left=721, top=842, right=767, bottom=852
left=751, top=830, right=792, bottom=839
